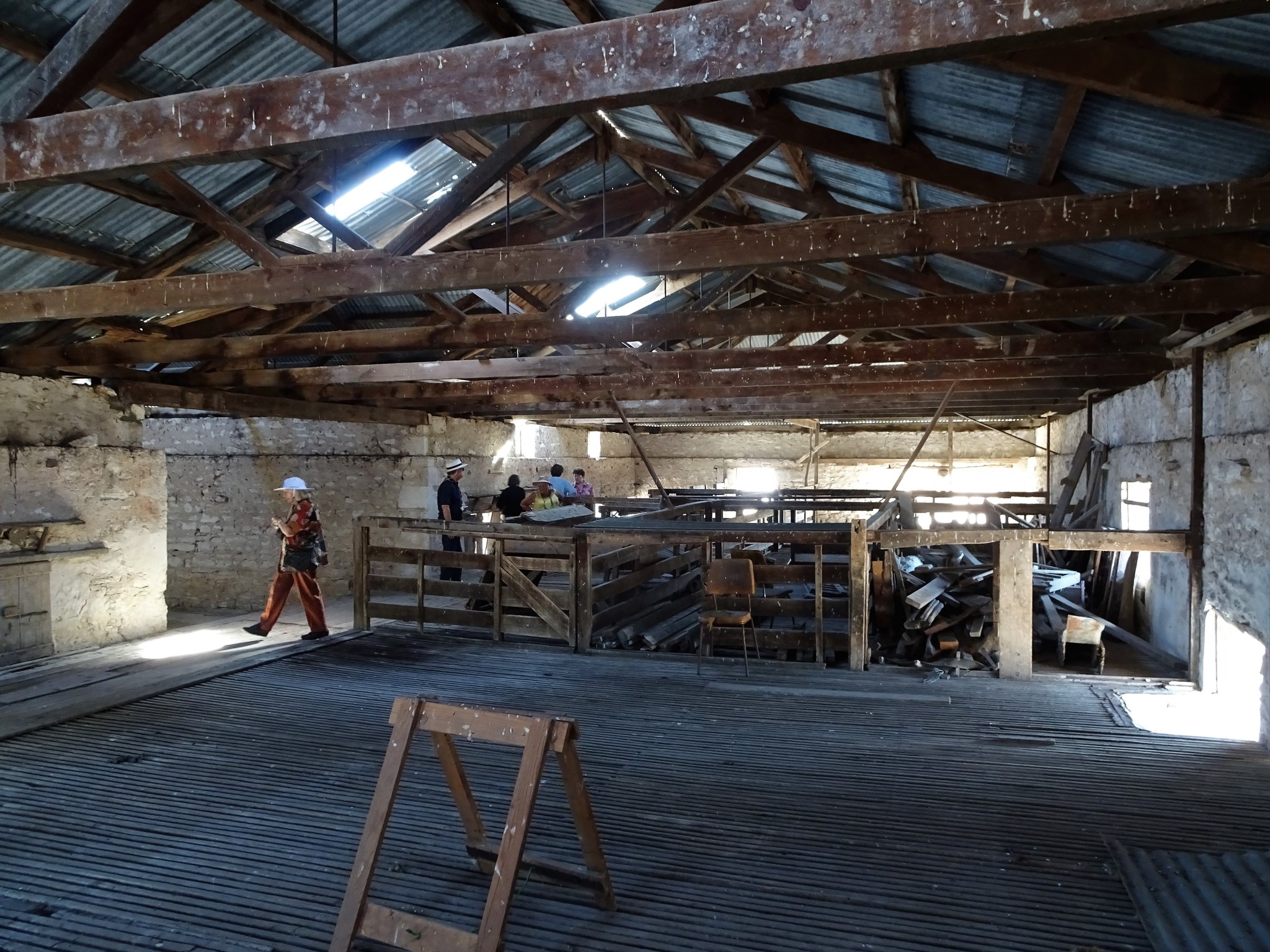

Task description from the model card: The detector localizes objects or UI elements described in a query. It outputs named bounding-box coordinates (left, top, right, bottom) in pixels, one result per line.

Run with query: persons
left=437, top=459, right=468, bottom=582
left=548, top=464, right=576, bottom=497
left=497, top=474, right=526, bottom=524
left=243, top=477, right=329, bottom=640
left=567, top=467, right=594, bottom=511
left=520, top=476, right=559, bottom=512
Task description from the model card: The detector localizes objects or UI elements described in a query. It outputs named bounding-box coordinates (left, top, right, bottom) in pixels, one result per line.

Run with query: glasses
left=457, top=468, right=464, bottom=471
left=544, top=501, right=553, bottom=509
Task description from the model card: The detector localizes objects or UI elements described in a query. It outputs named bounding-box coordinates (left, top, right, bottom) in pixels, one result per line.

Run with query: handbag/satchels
left=283, top=546, right=313, bottom=571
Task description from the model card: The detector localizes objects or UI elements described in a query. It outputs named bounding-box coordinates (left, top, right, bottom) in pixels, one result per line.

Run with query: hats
left=273, top=477, right=314, bottom=491
left=532, top=477, right=553, bottom=486
left=445, top=458, right=467, bottom=473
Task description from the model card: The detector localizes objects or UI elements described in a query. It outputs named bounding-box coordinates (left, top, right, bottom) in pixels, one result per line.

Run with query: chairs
left=698, top=559, right=760, bottom=677
left=731, top=549, right=795, bottom=628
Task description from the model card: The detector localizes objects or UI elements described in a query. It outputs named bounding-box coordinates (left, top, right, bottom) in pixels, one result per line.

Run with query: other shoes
left=243, top=623, right=269, bottom=637
left=301, top=629, right=329, bottom=640
left=543, top=571, right=547, bottom=576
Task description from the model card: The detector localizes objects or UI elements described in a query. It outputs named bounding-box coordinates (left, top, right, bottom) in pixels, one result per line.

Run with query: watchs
left=278, top=521, right=280, bottom=523
